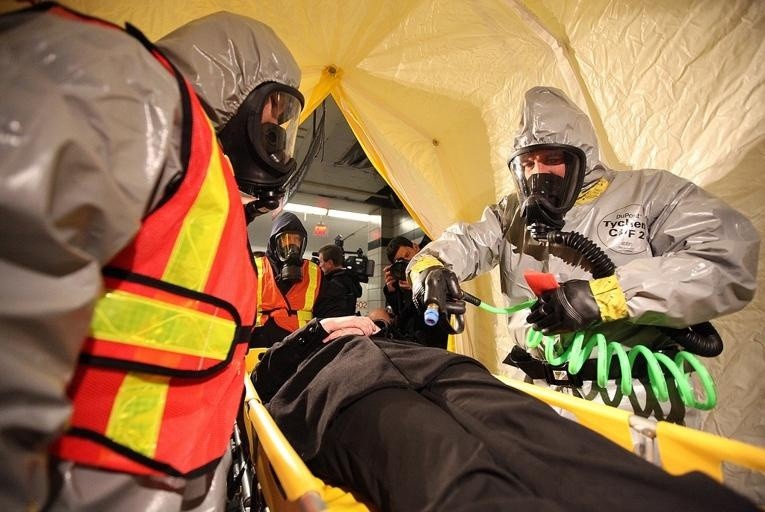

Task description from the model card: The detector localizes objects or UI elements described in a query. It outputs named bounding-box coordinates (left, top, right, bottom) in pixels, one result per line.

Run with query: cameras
left=391, top=257, right=410, bottom=281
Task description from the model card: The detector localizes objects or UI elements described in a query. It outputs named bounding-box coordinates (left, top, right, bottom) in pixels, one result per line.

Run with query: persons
left=406, top=86, right=760, bottom=421
left=250, top=315, right=765, bottom=512
left=384, top=236, right=450, bottom=350
left=318, top=244, right=362, bottom=314
left=250, top=213, right=360, bottom=348
left=0, top=0, right=306, bottom=512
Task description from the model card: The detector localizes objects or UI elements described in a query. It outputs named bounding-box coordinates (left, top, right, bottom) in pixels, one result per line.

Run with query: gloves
left=527, top=280, right=602, bottom=335
left=421, top=266, right=465, bottom=315
